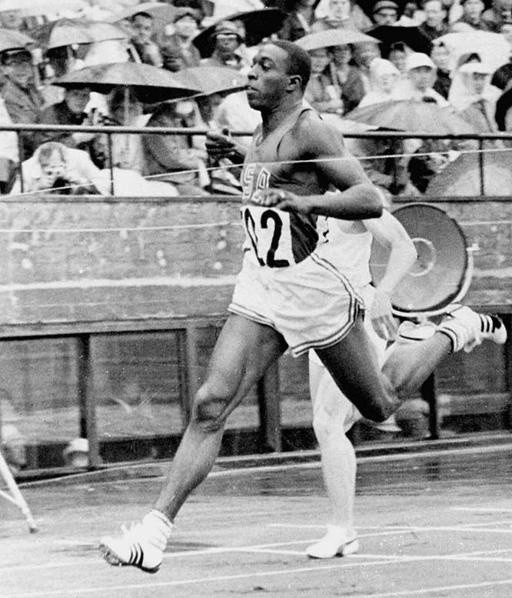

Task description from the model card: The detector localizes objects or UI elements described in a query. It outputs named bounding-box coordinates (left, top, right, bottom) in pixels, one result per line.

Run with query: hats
left=1, top=423, right=90, bottom=463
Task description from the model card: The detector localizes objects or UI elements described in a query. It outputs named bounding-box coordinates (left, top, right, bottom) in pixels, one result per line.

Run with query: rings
left=277, top=190, right=288, bottom=201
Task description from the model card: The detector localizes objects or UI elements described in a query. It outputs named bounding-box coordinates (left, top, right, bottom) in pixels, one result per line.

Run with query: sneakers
left=100, top=530, right=166, bottom=574
left=447, top=308, right=508, bottom=351
left=304, top=527, right=361, bottom=559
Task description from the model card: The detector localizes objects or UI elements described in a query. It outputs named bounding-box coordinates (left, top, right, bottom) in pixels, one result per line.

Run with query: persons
left=2, top=2, right=512, bottom=196
left=371, top=411, right=402, bottom=441
left=304, top=184, right=508, bottom=559
left=392, top=397, right=432, bottom=439
left=60, top=437, right=91, bottom=465
left=87, top=40, right=510, bottom=577
left=1, top=424, right=29, bottom=475
